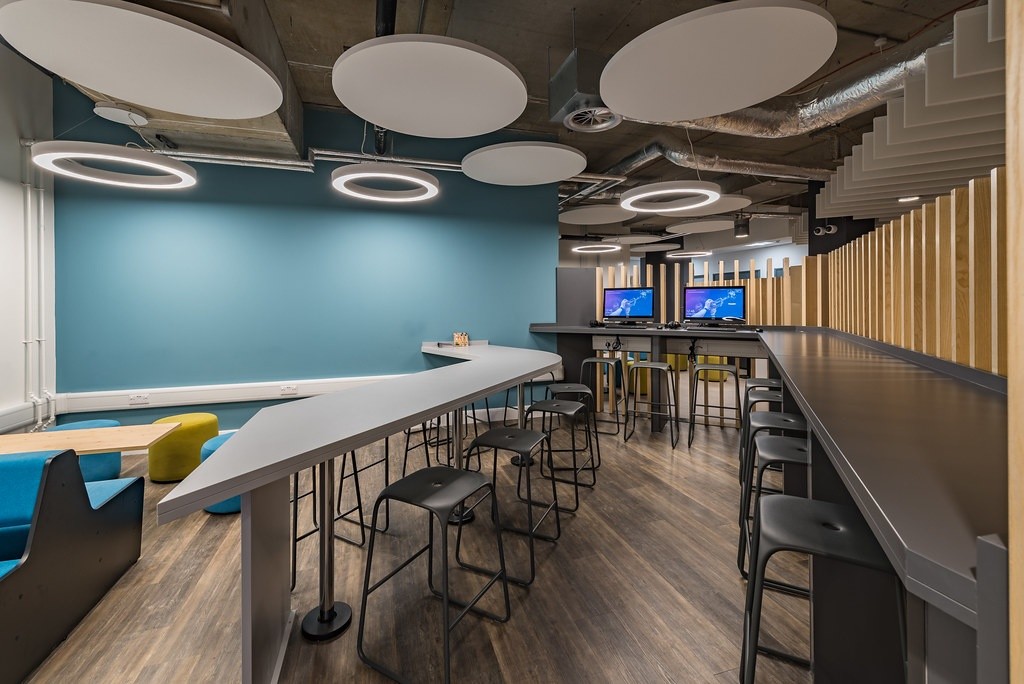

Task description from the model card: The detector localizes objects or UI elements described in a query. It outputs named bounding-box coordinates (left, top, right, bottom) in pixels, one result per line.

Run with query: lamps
left=620, top=181, right=721, bottom=212
left=666, top=249, right=711, bottom=258
left=330, top=162, right=439, bottom=203
left=30, top=102, right=198, bottom=189
left=734, top=187, right=749, bottom=238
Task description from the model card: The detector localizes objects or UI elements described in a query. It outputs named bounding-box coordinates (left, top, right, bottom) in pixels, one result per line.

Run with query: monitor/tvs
left=683, top=286, right=746, bottom=327
left=603, top=287, right=654, bottom=325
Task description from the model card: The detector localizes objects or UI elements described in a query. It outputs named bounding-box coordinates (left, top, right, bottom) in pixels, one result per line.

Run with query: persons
left=688, top=295, right=716, bottom=317
left=608, top=296, right=631, bottom=315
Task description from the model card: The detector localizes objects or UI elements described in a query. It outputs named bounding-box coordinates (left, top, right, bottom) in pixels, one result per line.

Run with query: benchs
left=0, top=448, right=145, bottom=684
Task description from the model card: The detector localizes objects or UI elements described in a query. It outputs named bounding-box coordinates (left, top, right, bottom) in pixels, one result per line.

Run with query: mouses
left=755, top=328, right=763, bottom=332
left=657, top=326, right=662, bottom=329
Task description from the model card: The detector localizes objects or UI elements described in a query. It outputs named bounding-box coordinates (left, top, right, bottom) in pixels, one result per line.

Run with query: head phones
left=590, top=320, right=605, bottom=327
left=665, top=321, right=681, bottom=329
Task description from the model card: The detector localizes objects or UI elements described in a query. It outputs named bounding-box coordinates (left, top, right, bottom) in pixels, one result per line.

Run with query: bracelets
left=620, top=306, right=624, bottom=310
left=704, top=307, right=709, bottom=311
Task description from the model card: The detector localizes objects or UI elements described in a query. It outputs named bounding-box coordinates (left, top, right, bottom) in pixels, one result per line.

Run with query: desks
left=529, top=322, right=1009, bottom=684
left=156, top=339, right=562, bottom=684
left=0, top=423, right=182, bottom=456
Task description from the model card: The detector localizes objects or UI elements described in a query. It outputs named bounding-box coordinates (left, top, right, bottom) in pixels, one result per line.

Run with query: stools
left=289, top=353, right=909, bottom=684
left=201, top=431, right=242, bottom=514
left=148, top=413, right=219, bottom=483
left=46, top=420, right=121, bottom=483
left=629, top=350, right=648, bottom=362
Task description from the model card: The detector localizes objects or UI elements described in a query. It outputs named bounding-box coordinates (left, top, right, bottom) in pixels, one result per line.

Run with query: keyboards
left=686, top=327, right=737, bottom=332
left=605, top=325, right=647, bottom=329
